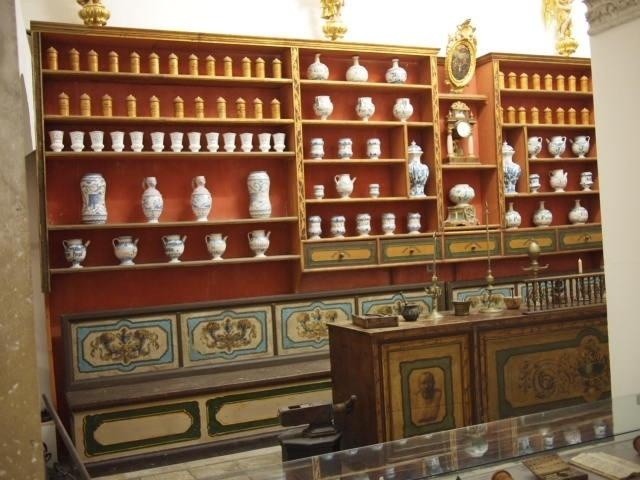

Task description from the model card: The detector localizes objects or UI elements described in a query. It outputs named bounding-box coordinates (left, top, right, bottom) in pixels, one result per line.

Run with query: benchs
left=48, top=277, right=450, bottom=479
left=447, top=267, right=605, bottom=311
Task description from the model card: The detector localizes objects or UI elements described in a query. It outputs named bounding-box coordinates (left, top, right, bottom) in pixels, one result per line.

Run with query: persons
left=417, top=371, right=441, bottom=423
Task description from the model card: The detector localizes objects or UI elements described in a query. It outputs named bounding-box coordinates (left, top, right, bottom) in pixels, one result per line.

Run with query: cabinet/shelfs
left=476, top=50, right=604, bottom=283
left=31, top=22, right=304, bottom=296
left=195, top=393, right=639, bottom=480
left=294, top=40, right=441, bottom=298
left=433, top=53, right=507, bottom=289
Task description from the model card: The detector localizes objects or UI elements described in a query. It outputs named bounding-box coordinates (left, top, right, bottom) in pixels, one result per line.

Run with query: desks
left=324, top=300, right=613, bottom=480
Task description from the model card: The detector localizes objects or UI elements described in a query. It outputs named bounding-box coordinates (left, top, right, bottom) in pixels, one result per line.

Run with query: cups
left=369, top=184, right=380, bottom=198
left=579, top=171, right=593, bottom=191
left=530, top=174, right=541, bottom=191
left=308, top=212, right=423, bottom=242
left=48, top=129, right=287, bottom=154
left=452, top=300, right=472, bottom=316
left=312, top=185, right=324, bottom=199
left=309, top=138, right=383, bottom=160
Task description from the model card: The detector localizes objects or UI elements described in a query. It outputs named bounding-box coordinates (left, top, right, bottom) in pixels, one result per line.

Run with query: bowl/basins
left=503, top=296, right=522, bottom=309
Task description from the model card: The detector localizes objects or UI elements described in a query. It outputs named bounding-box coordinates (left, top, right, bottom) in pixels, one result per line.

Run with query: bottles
left=46, top=45, right=288, bottom=120
left=504, top=141, right=520, bottom=192
left=532, top=201, right=554, bottom=226
left=498, top=71, right=589, bottom=124
left=567, top=199, right=589, bottom=225
left=140, top=176, right=164, bottom=223
left=307, top=52, right=415, bottom=122
left=78, top=173, right=110, bottom=226
left=189, top=175, right=212, bottom=220
left=506, top=201, right=522, bottom=228
left=403, top=304, right=420, bottom=322
left=248, top=171, right=271, bottom=217
left=409, top=139, right=429, bottom=198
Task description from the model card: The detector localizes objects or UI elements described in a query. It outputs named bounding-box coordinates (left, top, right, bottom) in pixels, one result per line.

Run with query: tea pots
left=548, top=168, right=568, bottom=192
left=61, top=228, right=273, bottom=268
left=569, top=135, right=592, bottom=157
left=528, top=135, right=543, bottom=159
left=546, top=136, right=566, bottom=158
left=334, top=173, right=357, bottom=198
